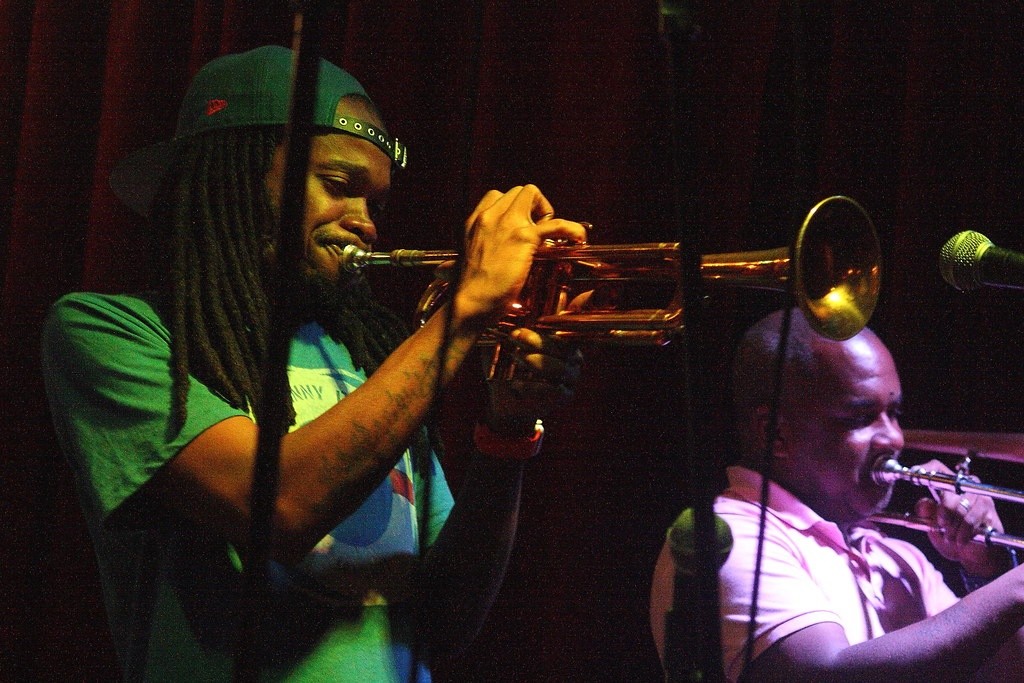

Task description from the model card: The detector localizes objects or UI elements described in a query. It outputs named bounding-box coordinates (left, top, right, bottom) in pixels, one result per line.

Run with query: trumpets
left=339, top=198, right=882, bottom=384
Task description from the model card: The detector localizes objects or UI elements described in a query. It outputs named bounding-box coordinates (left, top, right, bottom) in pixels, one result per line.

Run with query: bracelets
left=472, top=413, right=545, bottom=462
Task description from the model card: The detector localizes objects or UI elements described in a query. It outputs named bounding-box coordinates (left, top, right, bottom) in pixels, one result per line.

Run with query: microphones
left=940, top=229, right=1024, bottom=292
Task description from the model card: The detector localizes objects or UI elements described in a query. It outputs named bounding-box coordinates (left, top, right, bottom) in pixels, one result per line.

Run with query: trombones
left=840, top=427, right=1022, bottom=564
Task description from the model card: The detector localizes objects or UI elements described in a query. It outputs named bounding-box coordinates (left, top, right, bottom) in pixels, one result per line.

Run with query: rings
left=959, top=498, right=971, bottom=512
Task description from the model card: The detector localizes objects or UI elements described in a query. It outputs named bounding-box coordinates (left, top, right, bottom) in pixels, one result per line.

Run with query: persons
left=648, top=304, right=1024, bottom=682
left=39, top=41, right=592, bottom=681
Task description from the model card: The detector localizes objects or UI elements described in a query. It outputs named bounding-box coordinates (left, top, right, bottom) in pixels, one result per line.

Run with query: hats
left=173, top=45, right=409, bottom=167
